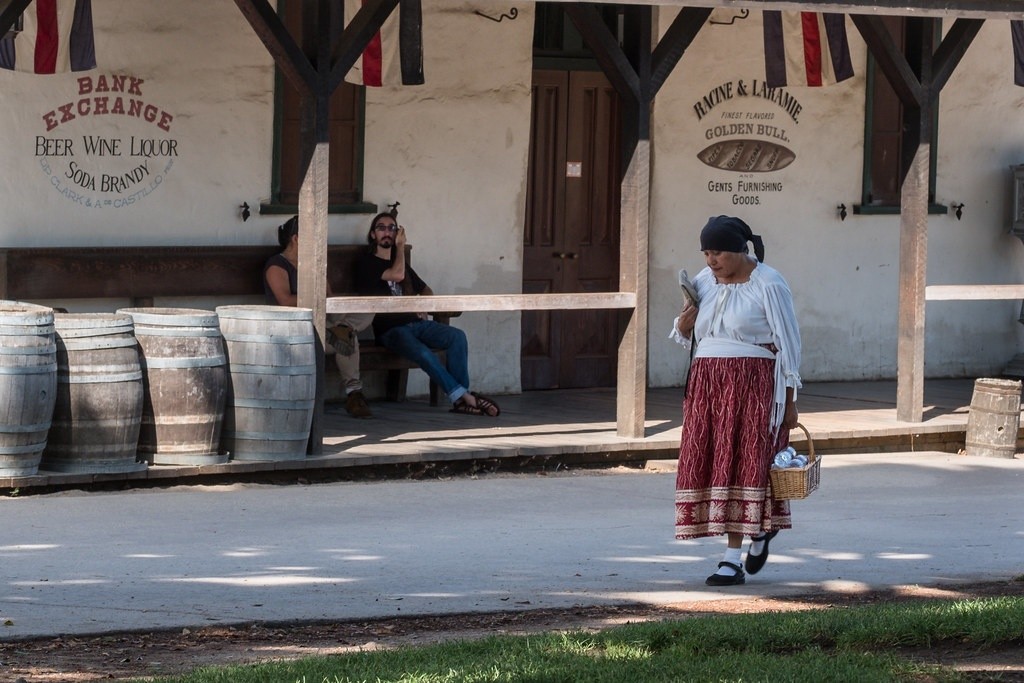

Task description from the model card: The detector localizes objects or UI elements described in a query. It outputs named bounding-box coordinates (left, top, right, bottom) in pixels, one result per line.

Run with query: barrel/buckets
left=212, top=305, right=317, bottom=462
left=114, top=306, right=224, bottom=458
left=965, top=377, right=1022, bottom=459
left=0, top=299, right=58, bottom=476
left=39, top=311, right=144, bottom=474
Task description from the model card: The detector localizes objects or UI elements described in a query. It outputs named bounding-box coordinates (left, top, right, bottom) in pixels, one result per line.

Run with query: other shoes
left=706, top=561, right=746, bottom=586
left=745, top=525, right=779, bottom=574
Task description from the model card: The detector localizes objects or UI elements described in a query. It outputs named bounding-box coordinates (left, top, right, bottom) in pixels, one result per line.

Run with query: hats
left=700, top=214, right=753, bottom=252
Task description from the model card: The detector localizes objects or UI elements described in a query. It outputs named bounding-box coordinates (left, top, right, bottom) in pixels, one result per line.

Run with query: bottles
left=771, top=446, right=810, bottom=469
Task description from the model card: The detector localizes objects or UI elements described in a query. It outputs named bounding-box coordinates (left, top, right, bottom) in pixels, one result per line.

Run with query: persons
left=263, top=214, right=377, bottom=417
left=350, top=212, right=502, bottom=417
left=666, top=214, right=804, bottom=586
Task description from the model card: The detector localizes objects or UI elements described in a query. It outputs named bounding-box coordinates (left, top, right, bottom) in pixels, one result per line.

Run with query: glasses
left=374, top=225, right=396, bottom=232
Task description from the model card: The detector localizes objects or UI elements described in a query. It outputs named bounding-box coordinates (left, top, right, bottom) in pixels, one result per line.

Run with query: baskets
left=769, top=422, right=822, bottom=501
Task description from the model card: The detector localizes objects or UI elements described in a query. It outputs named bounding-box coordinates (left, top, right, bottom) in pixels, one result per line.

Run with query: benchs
left=1, top=245, right=462, bottom=410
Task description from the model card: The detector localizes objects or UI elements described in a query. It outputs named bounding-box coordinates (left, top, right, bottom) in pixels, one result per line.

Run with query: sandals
left=448, top=391, right=500, bottom=417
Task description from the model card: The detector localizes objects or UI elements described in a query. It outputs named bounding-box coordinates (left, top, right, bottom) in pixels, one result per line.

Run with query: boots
left=345, top=390, right=372, bottom=418
left=325, top=323, right=355, bottom=356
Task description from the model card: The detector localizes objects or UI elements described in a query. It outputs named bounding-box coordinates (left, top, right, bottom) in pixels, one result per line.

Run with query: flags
left=0, top=0, right=99, bottom=75
left=1009, top=19, right=1024, bottom=88
left=343, top=0, right=427, bottom=87
left=762, top=9, right=856, bottom=89
